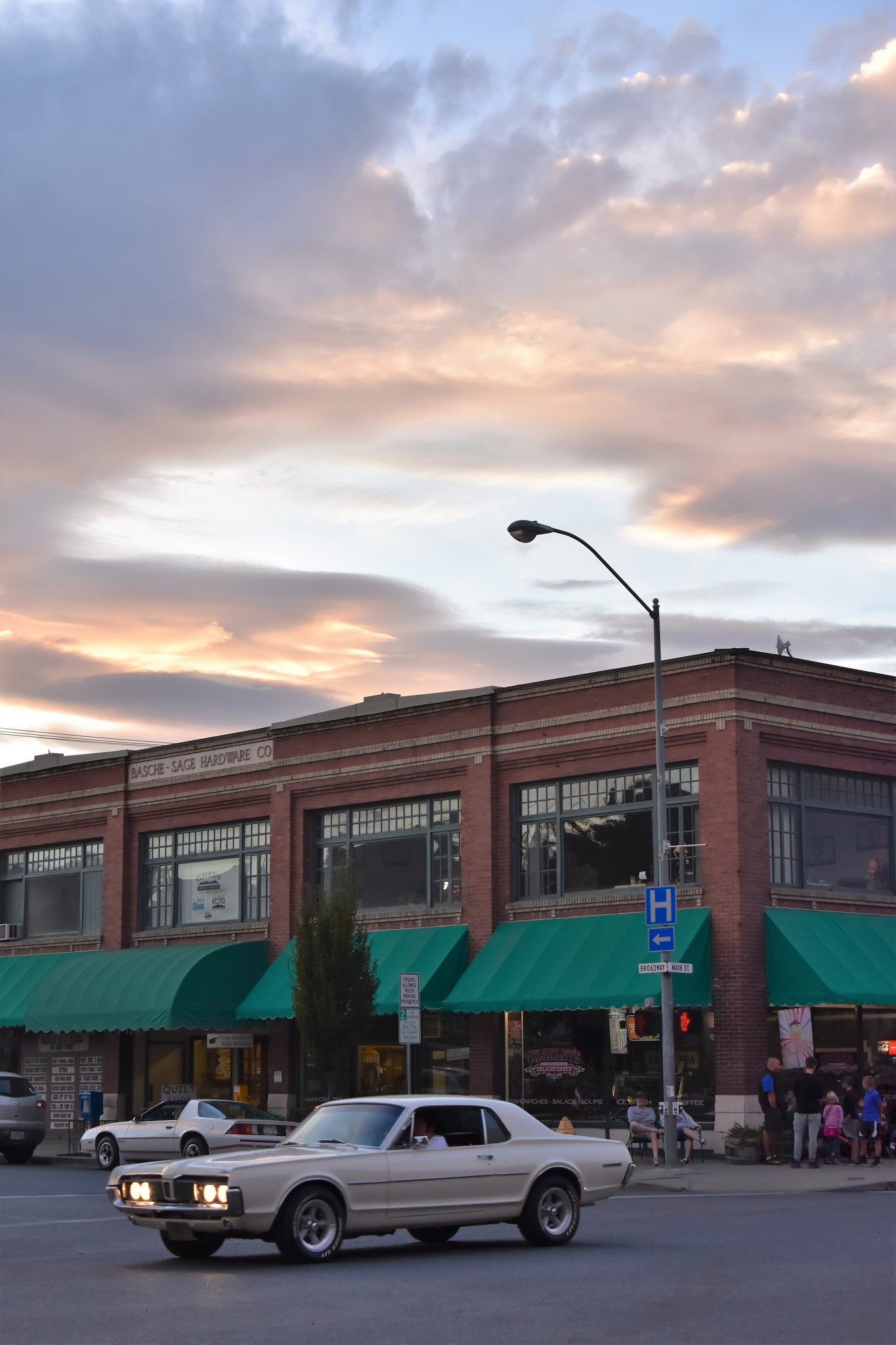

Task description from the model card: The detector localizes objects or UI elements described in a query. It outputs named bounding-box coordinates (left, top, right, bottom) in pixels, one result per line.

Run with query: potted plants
left=758, top=1122, right=795, bottom=1162
left=723, top=1121, right=763, bottom=1164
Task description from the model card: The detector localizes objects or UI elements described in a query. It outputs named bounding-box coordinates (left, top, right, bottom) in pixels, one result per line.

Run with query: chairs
left=442, top=1132, right=481, bottom=1147
left=786, top=1111, right=829, bottom=1161
left=625, top=1119, right=665, bottom=1164
left=658, top=1125, right=705, bottom=1164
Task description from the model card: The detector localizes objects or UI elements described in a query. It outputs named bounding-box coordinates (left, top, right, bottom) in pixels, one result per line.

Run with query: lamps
left=673, top=841, right=688, bottom=858
left=775, top=635, right=794, bottom=657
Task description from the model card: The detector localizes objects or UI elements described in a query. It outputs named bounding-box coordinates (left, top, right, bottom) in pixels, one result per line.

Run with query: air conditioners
left=0, top=923, right=22, bottom=940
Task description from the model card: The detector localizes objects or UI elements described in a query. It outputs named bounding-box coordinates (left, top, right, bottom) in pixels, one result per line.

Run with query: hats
left=787, top=1091, right=794, bottom=1100
left=635, top=1092, right=646, bottom=1099
left=837, top=1075, right=854, bottom=1085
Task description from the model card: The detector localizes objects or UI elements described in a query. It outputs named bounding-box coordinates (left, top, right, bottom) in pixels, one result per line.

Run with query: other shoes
left=833, top=1158, right=840, bottom=1165
left=853, top=1158, right=868, bottom=1166
left=773, top=1156, right=789, bottom=1162
left=870, top=1160, right=882, bottom=1167
left=789, top=1160, right=801, bottom=1168
left=889, top=1141, right=895, bottom=1149
left=842, top=1159, right=854, bottom=1166
left=766, top=1158, right=780, bottom=1165
left=824, top=1158, right=832, bottom=1163
left=808, top=1161, right=820, bottom=1169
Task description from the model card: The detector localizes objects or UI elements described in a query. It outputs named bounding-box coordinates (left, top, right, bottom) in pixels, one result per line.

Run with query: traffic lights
left=633, top=1009, right=661, bottom=1037
left=678, top=1007, right=702, bottom=1033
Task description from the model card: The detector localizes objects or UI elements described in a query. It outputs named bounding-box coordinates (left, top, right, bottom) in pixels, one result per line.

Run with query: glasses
left=637, top=1096, right=644, bottom=1098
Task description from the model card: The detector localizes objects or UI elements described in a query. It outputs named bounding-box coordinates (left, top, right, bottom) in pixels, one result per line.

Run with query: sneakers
left=653, top=1158, right=659, bottom=1166
left=658, top=1128, right=664, bottom=1135
left=699, top=1138, right=707, bottom=1145
left=680, top=1156, right=689, bottom=1165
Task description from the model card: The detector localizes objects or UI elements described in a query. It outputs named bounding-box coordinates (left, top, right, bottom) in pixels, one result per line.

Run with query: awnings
left=0, top=941, right=269, bottom=1032
left=763, top=905, right=896, bottom=1006
left=235, top=925, right=468, bottom=1020
left=444, top=907, right=713, bottom=1013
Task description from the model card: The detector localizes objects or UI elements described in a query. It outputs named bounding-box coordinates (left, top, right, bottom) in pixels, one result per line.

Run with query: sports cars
left=79, top=1099, right=301, bottom=1169
left=106, top=1094, right=636, bottom=1262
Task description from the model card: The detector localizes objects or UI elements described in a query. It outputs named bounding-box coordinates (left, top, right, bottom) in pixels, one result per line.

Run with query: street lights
left=505, top=520, right=682, bottom=1167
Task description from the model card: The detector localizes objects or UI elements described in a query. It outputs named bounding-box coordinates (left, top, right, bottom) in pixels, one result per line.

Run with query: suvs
left=0, top=1071, right=47, bottom=1166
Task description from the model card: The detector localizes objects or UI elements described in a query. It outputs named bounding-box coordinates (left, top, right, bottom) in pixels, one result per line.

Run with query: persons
left=659, top=1098, right=708, bottom=1164
left=410, top=1109, right=449, bottom=1151
left=759, top=1056, right=896, bottom=1169
left=627, top=1091, right=664, bottom=1165
